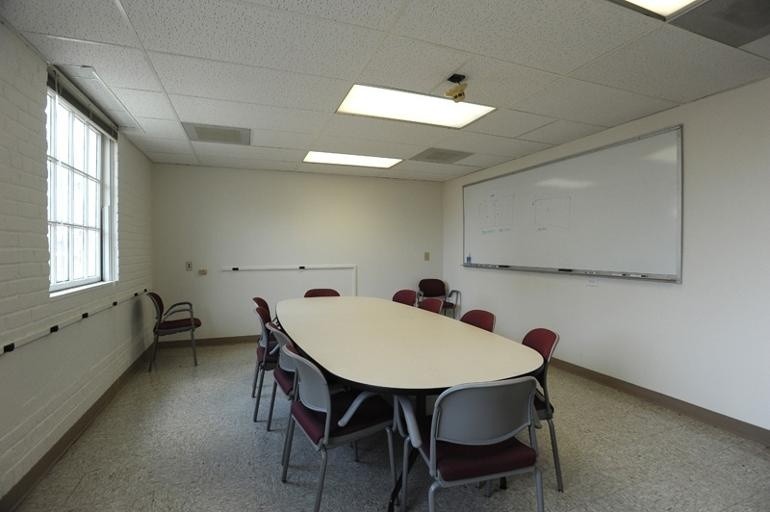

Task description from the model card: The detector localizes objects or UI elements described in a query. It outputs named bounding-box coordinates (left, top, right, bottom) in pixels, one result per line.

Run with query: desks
left=275, top=296, right=544, bottom=512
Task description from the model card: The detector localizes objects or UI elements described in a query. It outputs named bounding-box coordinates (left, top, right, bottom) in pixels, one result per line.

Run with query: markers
left=584, top=271, right=596, bottom=274
left=630, top=274, right=646, bottom=278
left=611, top=273, right=626, bottom=276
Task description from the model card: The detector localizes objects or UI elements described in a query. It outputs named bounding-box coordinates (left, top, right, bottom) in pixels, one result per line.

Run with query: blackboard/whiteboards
left=462, top=124, right=682, bottom=282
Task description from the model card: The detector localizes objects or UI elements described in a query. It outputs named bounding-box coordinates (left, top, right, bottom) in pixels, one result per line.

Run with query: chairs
left=395, top=376, right=544, bottom=512
left=392, top=290, right=416, bottom=306
left=459, top=310, right=496, bottom=332
left=146, top=291, right=206, bottom=372
left=416, top=297, right=444, bottom=314
left=304, top=288, right=341, bottom=298
left=417, top=279, right=458, bottom=319
left=265, top=322, right=353, bottom=466
left=522, top=328, right=562, bottom=492
left=281, top=344, right=405, bottom=512
left=252, top=297, right=279, bottom=423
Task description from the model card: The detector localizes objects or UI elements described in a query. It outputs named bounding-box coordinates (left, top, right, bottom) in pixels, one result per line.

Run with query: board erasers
left=498, top=265, right=510, bottom=268
left=558, top=269, right=572, bottom=271
